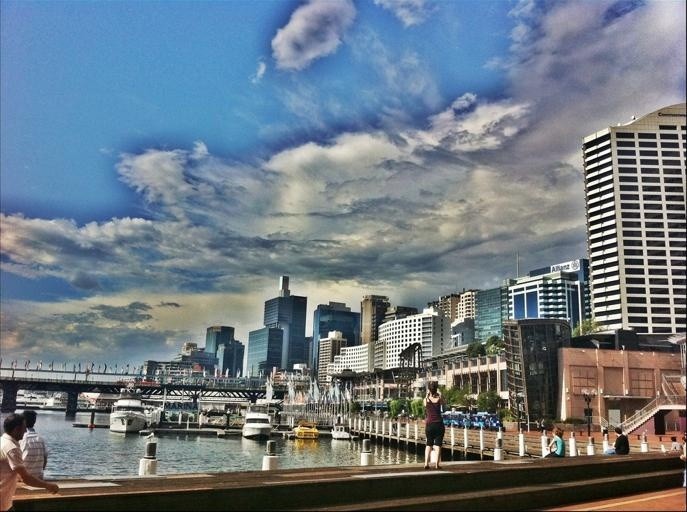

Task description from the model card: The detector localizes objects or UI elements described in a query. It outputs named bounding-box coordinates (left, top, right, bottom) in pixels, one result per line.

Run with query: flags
left=263, top=376, right=350, bottom=405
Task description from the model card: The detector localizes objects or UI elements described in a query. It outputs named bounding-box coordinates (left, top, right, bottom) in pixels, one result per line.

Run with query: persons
left=543, top=428, right=564, bottom=457
left=0, top=414, right=59, bottom=511
left=422, top=382, right=445, bottom=469
left=681, top=436, right=687, bottom=488
left=604, top=426, right=629, bottom=454
left=15, top=410, right=47, bottom=482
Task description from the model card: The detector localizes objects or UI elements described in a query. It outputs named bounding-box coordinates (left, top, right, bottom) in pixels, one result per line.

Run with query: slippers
left=424, top=464, right=441, bottom=470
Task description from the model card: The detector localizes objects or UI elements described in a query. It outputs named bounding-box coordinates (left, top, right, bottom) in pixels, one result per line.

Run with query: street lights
left=581, top=387, right=597, bottom=436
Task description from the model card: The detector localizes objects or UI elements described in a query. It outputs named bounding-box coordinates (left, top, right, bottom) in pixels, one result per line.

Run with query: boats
left=110, top=382, right=147, bottom=433
left=331, top=424, right=350, bottom=439
left=242, top=413, right=272, bottom=439
left=294, top=420, right=319, bottom=439
